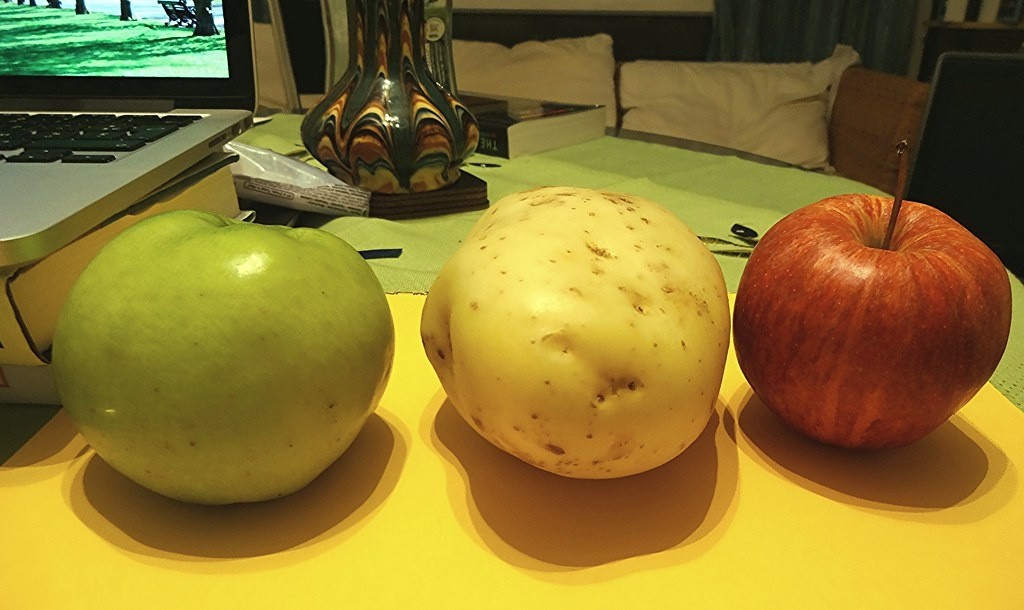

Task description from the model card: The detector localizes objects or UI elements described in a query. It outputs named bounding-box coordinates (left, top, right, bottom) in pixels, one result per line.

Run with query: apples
left=733, top=139, right=1012, bottom=455
left=51, top=208, right=397, bottom=505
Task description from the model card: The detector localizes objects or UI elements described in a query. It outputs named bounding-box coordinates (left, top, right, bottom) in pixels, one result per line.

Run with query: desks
left=0, top=127, right=1024, bottom=610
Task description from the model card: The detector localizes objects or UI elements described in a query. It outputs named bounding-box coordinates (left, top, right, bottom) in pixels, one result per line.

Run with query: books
left=456, top=91, right=607, bottom=160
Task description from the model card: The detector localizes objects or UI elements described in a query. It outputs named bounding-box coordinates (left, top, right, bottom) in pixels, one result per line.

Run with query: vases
left=300, top=0, right=479, bottom=195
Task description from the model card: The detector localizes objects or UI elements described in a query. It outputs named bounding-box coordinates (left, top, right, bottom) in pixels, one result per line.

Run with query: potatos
left=417, top=185, right=730, bottom=480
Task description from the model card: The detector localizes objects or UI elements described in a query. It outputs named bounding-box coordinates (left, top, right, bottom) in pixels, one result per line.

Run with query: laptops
left=0, top=0, right=257, bottom=267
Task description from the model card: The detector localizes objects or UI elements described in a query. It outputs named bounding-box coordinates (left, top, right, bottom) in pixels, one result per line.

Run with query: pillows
left=439, top=31, right=619, bottom=133
left=619, top=60, right=830, bottom=171
